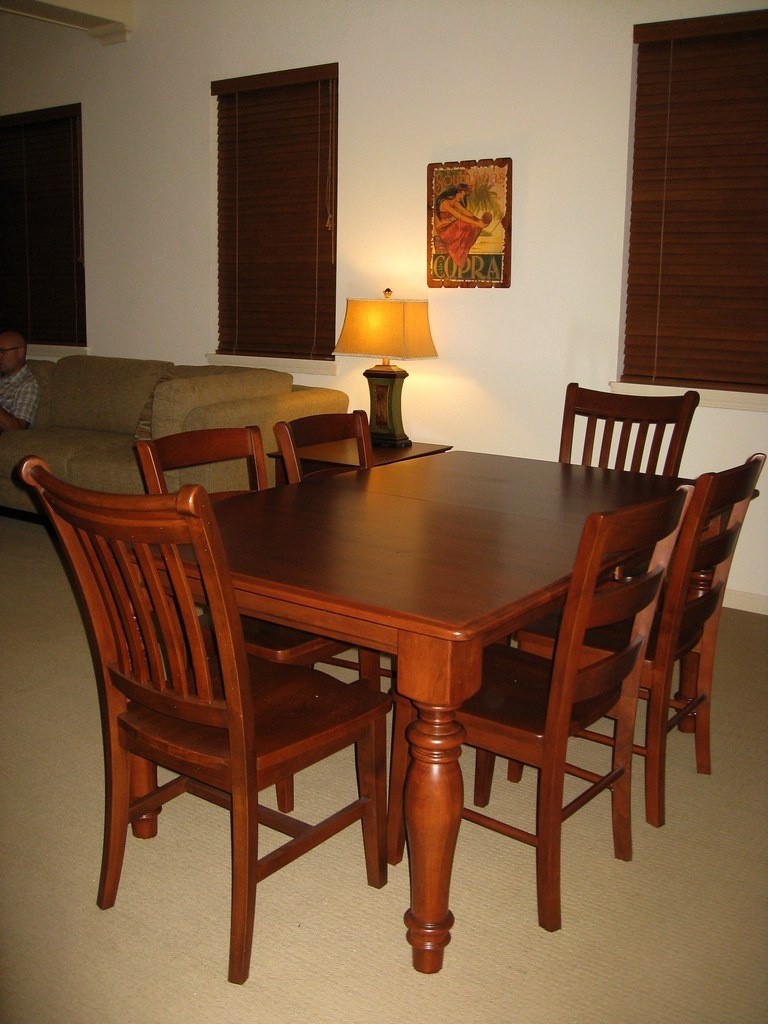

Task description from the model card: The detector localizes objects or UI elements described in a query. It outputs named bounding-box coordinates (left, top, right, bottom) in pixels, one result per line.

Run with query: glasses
left=0, top=347, right=20, bottom=354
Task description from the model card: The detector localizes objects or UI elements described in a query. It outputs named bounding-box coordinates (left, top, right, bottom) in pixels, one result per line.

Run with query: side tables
left=265, top=437, right=454, bottom=486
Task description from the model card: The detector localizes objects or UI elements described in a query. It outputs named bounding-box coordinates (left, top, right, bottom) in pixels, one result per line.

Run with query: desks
left=126, top=450, right=759, bottom=975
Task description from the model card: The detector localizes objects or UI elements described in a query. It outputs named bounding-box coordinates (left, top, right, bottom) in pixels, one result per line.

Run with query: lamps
left=331, top=287, right=440, bottom=450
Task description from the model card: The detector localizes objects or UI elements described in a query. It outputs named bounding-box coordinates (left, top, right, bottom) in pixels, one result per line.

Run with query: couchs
left=0, top=353, right=349, bottom=526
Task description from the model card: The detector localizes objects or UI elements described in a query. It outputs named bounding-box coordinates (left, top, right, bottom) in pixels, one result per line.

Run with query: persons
left=0, top=331, right=41, bottom=432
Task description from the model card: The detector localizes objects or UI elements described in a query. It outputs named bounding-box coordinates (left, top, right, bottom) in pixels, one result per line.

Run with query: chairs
left=135, top=425, right=355, bottom=815
left=559, top=381, right=700, bottom=582
left=507, top=453, right=767, bottom=828
left=273, top=409, right=374, bottom=484
left=385, top=484, right=696, bottom=932
left=14, top=454, right=393, bottom=986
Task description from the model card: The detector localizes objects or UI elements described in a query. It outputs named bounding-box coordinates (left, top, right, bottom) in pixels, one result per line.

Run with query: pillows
left=133, top=373, right=171, bottom=440
